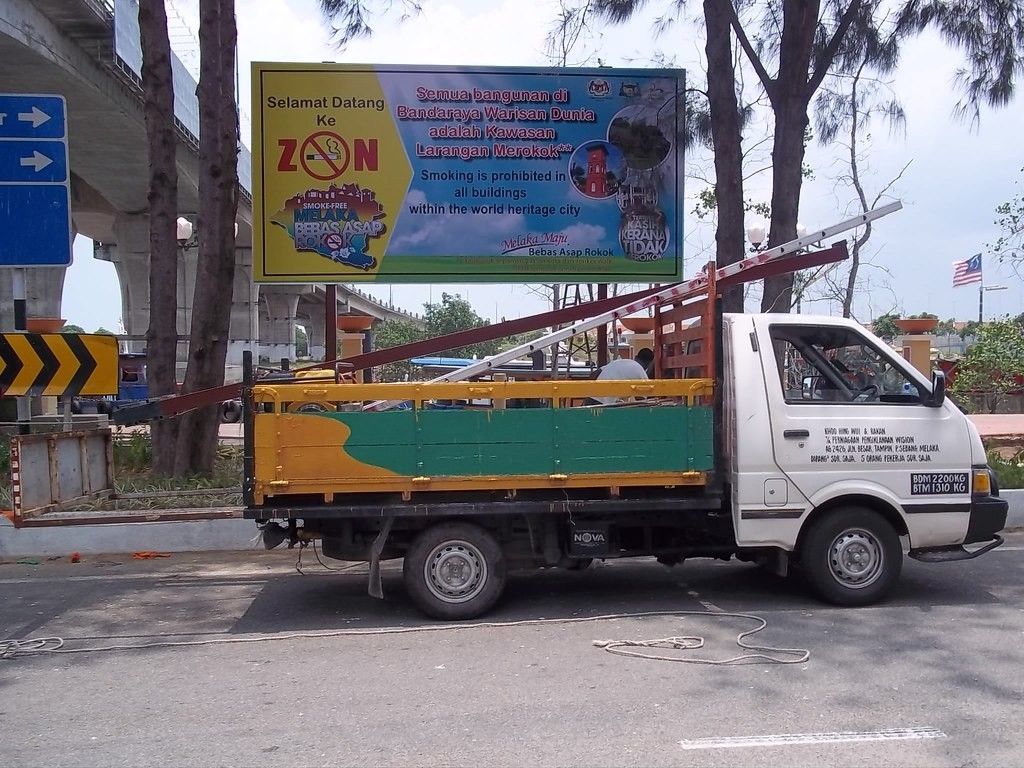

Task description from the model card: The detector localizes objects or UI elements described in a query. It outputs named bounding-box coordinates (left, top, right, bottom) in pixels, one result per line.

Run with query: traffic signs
left=0, top=94, right=73, bottom=267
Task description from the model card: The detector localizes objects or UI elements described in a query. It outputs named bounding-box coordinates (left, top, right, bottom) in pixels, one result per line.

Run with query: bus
left=405, top=357, right=595, bottom=382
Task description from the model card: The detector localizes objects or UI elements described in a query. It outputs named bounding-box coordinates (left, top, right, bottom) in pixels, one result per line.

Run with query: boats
left=58, top=352, right=147, bottom=414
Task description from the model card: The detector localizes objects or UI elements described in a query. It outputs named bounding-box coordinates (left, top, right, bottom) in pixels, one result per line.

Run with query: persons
left=584, top=348, right=652, bottom=406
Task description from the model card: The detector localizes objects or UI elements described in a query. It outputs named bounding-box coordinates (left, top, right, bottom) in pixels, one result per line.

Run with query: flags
left=953, top=252, right=982, bottom=288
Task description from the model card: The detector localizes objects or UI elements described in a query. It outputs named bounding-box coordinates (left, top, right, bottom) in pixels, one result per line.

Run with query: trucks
left=240, top=261, right=1008, bottom=621
